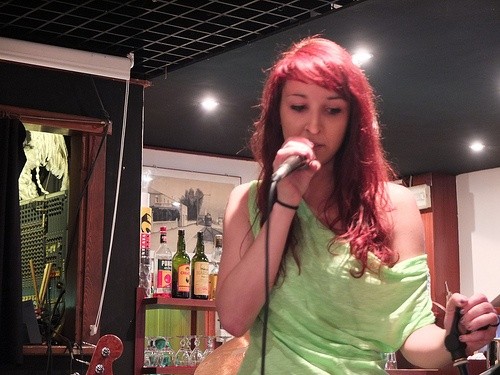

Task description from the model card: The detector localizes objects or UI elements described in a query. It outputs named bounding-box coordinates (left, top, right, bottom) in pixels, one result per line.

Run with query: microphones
left=271, top=154, right=307, bottom=180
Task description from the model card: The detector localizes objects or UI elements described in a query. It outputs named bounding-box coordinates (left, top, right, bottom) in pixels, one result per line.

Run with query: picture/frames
left=137, top=164, right=243, bottom=279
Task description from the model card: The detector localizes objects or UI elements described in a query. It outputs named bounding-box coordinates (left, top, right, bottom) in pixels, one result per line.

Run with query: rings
left=490, top=317, right=500, bottom=327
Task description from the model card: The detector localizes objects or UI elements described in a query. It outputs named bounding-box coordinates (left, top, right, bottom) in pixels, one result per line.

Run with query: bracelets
left=276, top=200, right=298, bottom=210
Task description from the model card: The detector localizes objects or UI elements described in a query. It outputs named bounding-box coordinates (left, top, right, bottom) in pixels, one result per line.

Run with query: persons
left=216, top=37, right=498, bottom=375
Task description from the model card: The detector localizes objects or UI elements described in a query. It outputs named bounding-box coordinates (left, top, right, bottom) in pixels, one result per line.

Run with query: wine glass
left=218, top=335, right=232, bottom=345
left=143, top=334, right=193, bottom=367
left=188, top=334, right=205, bottom=367
left=203, top=335, right=217, bottom=356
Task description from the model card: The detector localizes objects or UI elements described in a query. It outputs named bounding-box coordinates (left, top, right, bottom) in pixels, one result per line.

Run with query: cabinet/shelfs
left=138, top=298, right=216, bottom=375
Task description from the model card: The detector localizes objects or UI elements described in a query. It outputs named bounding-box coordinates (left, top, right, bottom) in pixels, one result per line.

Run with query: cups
left=385, top=352, right=397, bottom=370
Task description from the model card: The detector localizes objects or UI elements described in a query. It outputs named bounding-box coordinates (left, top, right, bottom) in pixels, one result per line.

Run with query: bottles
left=171, top=229, right=191, bottom=299
left=152, top=226, right=172, bottom=298
left=191, top=232, right=209, bottom=300
left=208, top=235, right=223, bottom=301
